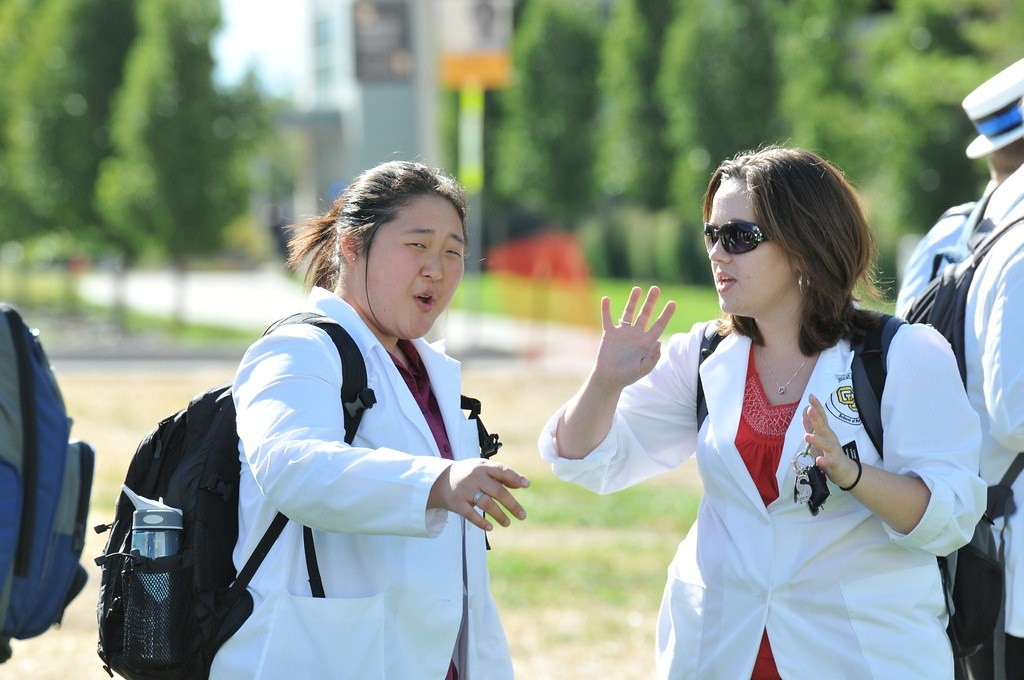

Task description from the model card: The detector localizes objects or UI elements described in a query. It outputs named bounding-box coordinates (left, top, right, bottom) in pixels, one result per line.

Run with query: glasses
left=700, top=220, right=767, bottom=253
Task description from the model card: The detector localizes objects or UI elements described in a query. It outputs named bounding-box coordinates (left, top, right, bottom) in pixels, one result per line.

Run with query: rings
left=472, top=491, right=485, bottom=504
left=619, top=320, right=632, bottom=325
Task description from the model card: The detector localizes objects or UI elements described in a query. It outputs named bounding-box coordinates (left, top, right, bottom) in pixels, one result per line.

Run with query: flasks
left=123, top=509, right=184, bottom=666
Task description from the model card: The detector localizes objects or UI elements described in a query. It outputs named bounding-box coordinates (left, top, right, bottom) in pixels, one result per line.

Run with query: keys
left=793, top=449, right=832, bottom=518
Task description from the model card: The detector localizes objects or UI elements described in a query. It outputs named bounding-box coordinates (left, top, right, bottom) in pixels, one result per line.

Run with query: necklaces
left=756, top=341, right=808, bottom=395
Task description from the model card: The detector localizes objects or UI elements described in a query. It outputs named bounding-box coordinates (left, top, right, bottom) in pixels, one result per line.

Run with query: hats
left=959, top=57, right=1024, bottom=158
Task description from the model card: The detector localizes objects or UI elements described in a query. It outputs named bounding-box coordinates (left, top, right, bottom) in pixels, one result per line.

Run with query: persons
left=205, top=160, right=531, bottom=678
left=892, top=62, right=1024, bottom=680
left=539, top=146, right=983, bottom=680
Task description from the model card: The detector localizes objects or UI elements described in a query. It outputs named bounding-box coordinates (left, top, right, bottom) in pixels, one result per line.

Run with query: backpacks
left=697, top=311, right=1003, bottom=661
left=0, top=297, right=93, bottom=665
left=904, top=180, right=1024, bottom=518
left=96, top=310, right=500, bottom=679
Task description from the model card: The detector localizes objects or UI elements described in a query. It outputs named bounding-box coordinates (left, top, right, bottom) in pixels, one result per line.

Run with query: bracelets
left=838, top=458, right=863, bottom=492
